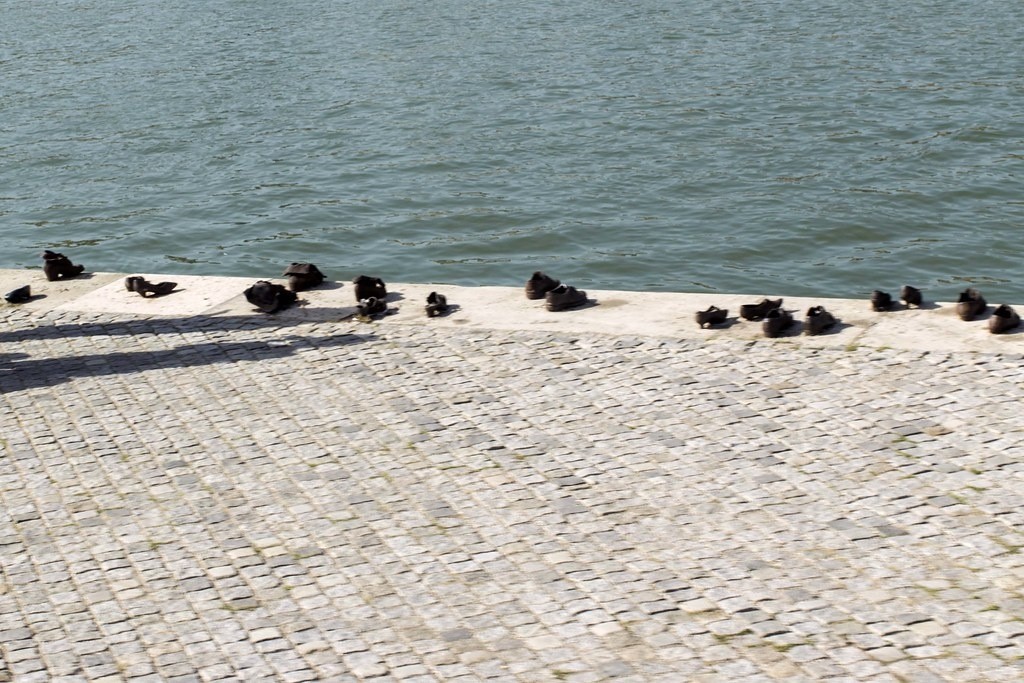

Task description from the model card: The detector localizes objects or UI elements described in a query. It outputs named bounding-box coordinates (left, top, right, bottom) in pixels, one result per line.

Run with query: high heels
left=42, top=250, right=84, bottom=281
left=696, top=306, right=728, bottom=328
left=125, top=276, right=177, bottom=298
left=425, top=292, right=446, bottom=317
left=871, top=290, right=891, bottom=312
left=740, top=299, right=782, bottom=320
left=900, top=286, right=921, bottom=309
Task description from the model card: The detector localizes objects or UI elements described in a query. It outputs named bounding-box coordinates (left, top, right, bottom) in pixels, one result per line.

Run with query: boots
left=354, top=275, right=387, bottom=302
left=281, top=263, right=324, bottom=291
left=244, top=281, right=298, bottom=313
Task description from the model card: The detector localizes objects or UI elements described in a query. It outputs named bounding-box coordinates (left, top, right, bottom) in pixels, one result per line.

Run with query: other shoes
left=5, top=285, right=30, bottom=303
left=990, top=304, right=1021, bottom=334
left=356, top=297, right=386, bottom=316
left=957, top=288, right=985, bottom=321
left=526, top=271, right=587, bottom=311
left=764, top=309, right=793, bottom=337
left=805, top=306, right=836, bottom=336
